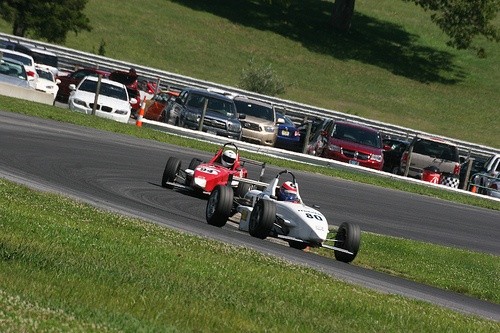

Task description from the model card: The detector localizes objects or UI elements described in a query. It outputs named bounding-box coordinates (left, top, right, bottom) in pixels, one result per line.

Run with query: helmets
left=221, top=149, right=237, bottom=167
left=280, top=181, right=298, bottom=200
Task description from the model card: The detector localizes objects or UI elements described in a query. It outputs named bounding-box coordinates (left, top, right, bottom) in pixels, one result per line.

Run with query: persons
left=277, top=181, right=298, bottom=201
left=221, top=150, right=236, bottom=168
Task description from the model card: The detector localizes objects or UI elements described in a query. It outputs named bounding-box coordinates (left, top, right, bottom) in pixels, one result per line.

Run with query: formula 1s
left=206, top=162, right=362, bottom=264
left=161, top=143, right=248, bottom=196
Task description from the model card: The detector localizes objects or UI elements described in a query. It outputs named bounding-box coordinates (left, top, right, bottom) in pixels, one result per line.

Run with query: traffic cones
left=135, top=95, right=146, bottom=127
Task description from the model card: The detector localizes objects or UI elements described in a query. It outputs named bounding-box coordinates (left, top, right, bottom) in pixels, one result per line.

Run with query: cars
left=0, top=41, right=140, bottom=115
left=164, top=87, right=246, bottom=140
left=68, top=76, right=138, bottom=124
left=308, top=118, right=392, bottom=172
left=233, top=95, right=320, bottom=150
left=141, top=83, right=180, bottom=122
left=383, top=134, right=500, bottom=199
